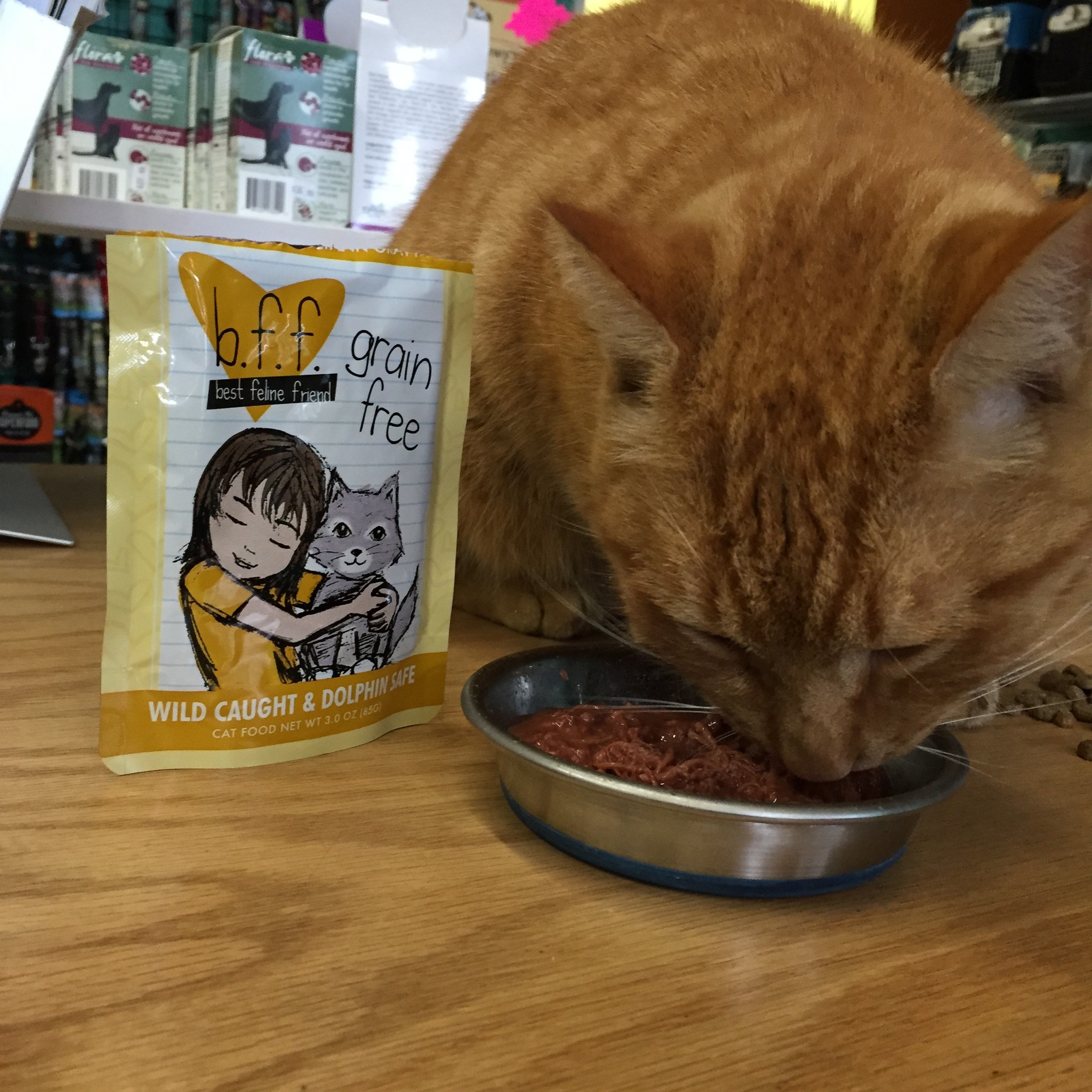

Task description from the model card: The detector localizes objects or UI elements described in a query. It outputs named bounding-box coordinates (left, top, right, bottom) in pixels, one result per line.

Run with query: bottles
left=0, top=232, right=106, bottom=464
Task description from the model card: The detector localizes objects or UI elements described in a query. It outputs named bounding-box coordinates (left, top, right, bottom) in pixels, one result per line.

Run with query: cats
left=383, top=0, right=1092, bottom=781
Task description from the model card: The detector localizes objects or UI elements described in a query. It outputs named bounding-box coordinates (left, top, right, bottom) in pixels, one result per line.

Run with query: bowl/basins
left=459, top=640, right=968, bottom=896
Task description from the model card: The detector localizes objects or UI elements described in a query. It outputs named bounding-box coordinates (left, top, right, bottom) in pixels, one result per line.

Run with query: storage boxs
left=33, top=0, right=492, bottom=232
